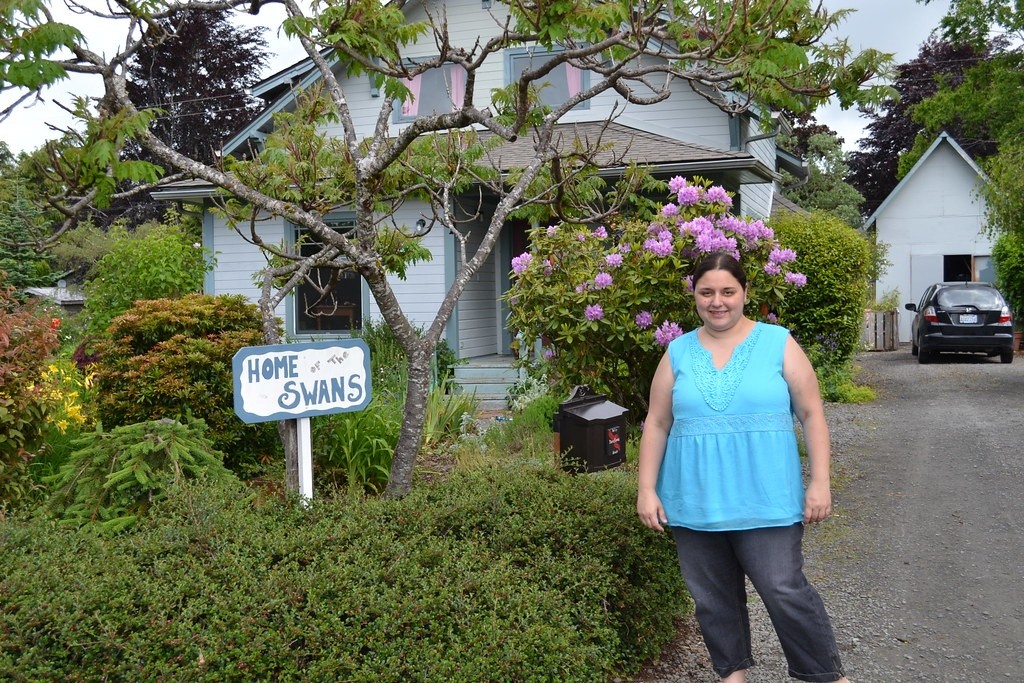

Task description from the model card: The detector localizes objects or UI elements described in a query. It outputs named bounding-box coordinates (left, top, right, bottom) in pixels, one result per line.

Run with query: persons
left=636, top=255, right=847, bottom=683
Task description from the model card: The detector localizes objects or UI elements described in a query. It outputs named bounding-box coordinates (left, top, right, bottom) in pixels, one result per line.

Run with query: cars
left=904, top=280, right=1015, bottom=365
